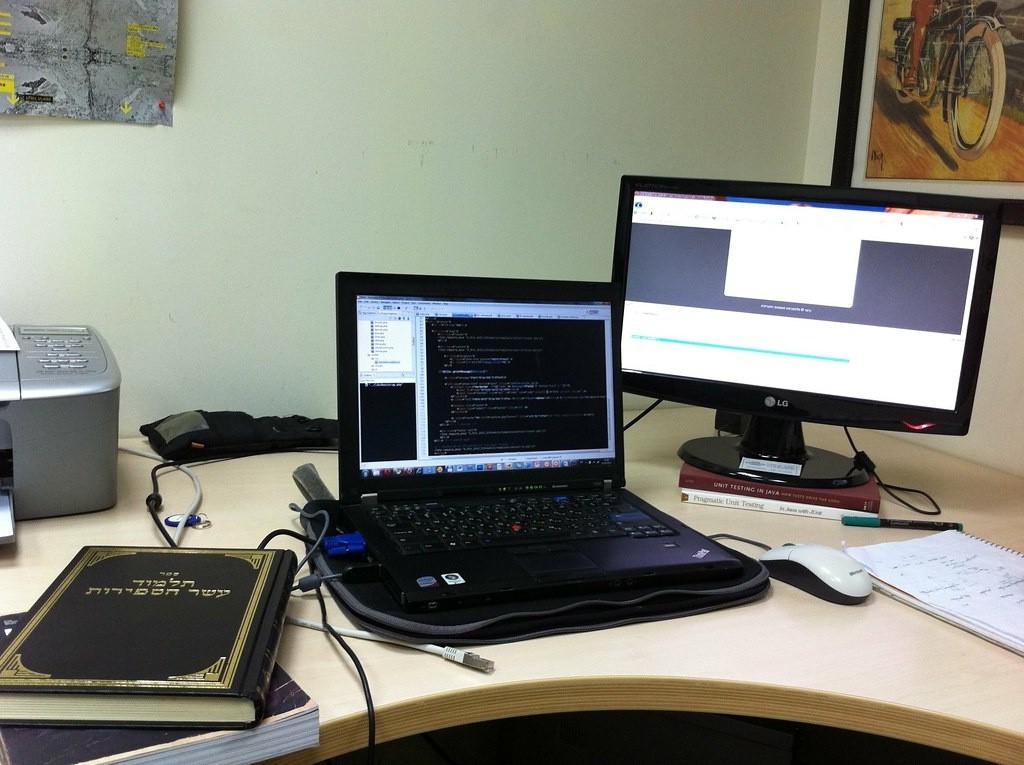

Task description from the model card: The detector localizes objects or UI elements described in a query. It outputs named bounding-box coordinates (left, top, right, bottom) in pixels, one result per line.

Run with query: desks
left=0, top=407, right=1024, bottom=765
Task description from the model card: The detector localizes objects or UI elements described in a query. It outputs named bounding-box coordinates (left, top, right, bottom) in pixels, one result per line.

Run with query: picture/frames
left=827, top=0, right=1024, bottom=226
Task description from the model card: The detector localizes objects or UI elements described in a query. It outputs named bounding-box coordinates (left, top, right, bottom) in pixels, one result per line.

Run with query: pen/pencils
left=841, top=516, right=963, bottom=533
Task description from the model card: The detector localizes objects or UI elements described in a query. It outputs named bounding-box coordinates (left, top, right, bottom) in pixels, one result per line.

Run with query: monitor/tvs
left=610, top=174, right=1007, bottom=489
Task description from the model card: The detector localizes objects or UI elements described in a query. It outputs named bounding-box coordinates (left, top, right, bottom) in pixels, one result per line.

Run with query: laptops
left=334, top=272, right=743, bottom=609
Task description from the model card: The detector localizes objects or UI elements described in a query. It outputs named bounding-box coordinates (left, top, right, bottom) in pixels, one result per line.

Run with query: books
left=678, top=460, right=881, bottom=522
left=0, top=545, right=319, bottom=765
left=839, top=529, right=1024, bottom=658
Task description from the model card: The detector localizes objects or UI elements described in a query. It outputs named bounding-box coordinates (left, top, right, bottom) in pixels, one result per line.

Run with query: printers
left=0, top=323, right=124, bottom=544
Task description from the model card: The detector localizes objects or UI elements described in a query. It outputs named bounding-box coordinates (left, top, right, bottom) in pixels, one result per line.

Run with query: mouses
left=760, top=542, right=873, bottom=607
